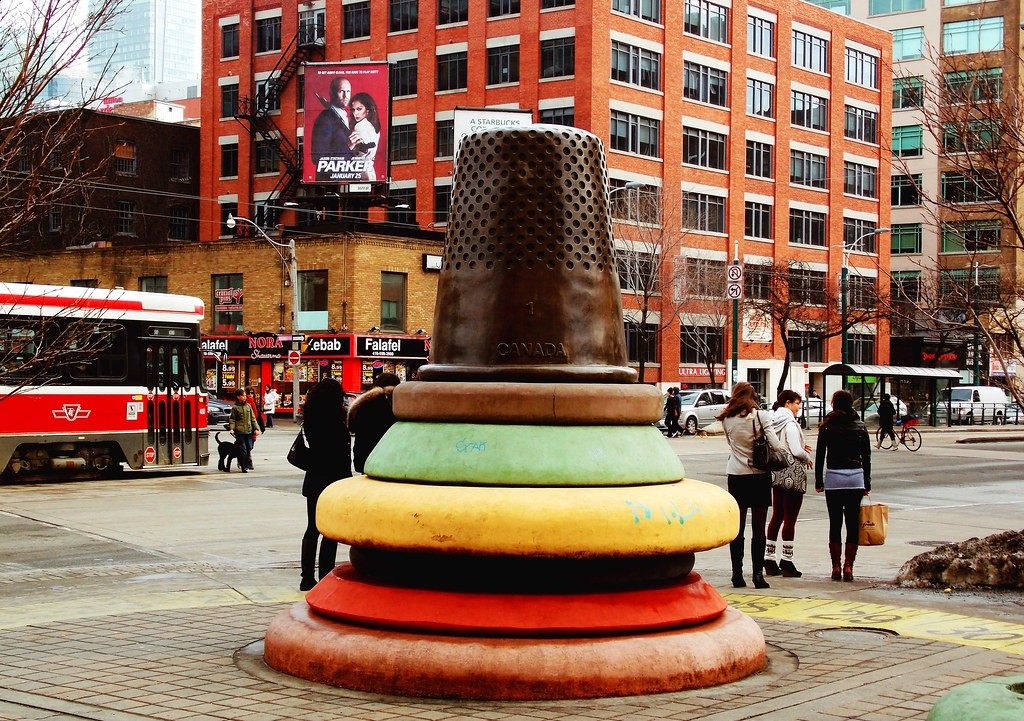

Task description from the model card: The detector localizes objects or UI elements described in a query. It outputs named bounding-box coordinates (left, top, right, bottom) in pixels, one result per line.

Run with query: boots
left=752, top=537, right=770, bottom=588
left=873, top=439, right=883, bottom=449
left=843, top=542, right=857, bottom=581
left=891, top=440, right=898, bottom=450
left=830, top=541, right=842, bottom=579
left=319, top=540, right=337, bottom=579
left=300, top=539, right=318, bottom=590
left=730, top=537, right=746, bottom=588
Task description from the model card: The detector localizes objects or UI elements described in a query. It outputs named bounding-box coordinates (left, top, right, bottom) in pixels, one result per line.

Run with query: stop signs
left=288, top=350, right=300, bottom=366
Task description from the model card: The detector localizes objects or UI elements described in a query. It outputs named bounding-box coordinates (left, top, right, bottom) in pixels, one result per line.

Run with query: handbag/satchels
left=770, top=450, right=807, bottom=494
left=844, top=494, right=889, bottom=545
left=287, top=425, right=311, bottom=470
left=264, top=404, right=272, bottom=410
left=751, top=410, right=790, bottom=472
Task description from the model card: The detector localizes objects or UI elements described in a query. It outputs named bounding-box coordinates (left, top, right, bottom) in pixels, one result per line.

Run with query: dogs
left=214, top=429, right=240, bottom=472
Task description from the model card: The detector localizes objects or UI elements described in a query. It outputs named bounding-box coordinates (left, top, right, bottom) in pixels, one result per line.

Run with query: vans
left=926, top=386, right=1009, bottom=425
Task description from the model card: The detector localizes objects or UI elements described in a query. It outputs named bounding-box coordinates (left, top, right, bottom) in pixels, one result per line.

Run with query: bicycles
left=876, top=421, right=922, bottom=452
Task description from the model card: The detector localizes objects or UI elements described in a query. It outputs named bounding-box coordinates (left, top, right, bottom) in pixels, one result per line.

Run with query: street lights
left=841, top=227, right=891, bottom=390
left=227, top=213, right=300, bottom=424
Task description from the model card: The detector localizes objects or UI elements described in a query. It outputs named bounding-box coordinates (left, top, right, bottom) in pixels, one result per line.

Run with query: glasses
left=247, top=391, right=254, bottom=395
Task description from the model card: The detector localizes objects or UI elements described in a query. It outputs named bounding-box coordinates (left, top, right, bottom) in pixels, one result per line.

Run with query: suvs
left=654, top=388, right=732, bottom=434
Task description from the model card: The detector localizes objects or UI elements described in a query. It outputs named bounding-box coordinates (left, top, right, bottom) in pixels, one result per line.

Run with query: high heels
left=778, top=558, right=802, bottom=577
left=764, top=560, right=781, bottom=575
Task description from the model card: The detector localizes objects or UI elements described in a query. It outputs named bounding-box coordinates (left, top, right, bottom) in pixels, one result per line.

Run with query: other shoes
left=681, top=430, right=685, bottom=436
left=672, top=431, right=678, bottom=437
left=243, top=468, right=249, bottom=473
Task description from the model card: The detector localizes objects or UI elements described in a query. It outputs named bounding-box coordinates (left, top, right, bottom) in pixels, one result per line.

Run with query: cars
left=1006, top=403, right=1024, bottom=424
left=852, top=395, right=908, bottom=427
left=795, top=398, right=834, bottom=429
left=207, top=391, right=232, bottom=425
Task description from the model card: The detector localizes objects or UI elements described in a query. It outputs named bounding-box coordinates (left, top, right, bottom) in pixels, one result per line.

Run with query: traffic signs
left=292, top=334, right=306, bottom=343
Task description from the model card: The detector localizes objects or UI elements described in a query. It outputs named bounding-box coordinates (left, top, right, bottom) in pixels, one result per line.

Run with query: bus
left=0, top=281, right=228, bottom=485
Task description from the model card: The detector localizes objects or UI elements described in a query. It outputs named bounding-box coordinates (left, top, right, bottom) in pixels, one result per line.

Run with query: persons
left=762, top=390, right=814, bottom=577
left=664, top=387, right=685, bottom=438
left=348, top=372, right=401, bottom=475
left=299, top=379, right=353, bottom=591
left=311, top=77, right=380, bottom=181
left=263, top=384, right=275, bottom=428
left=229, top=386, right=265, bottom=473
left=874, top=394, right=898, bottom=451
left=815, top=391, right=872, bottom=581
left=715, top=382, right=780, bottom=588
